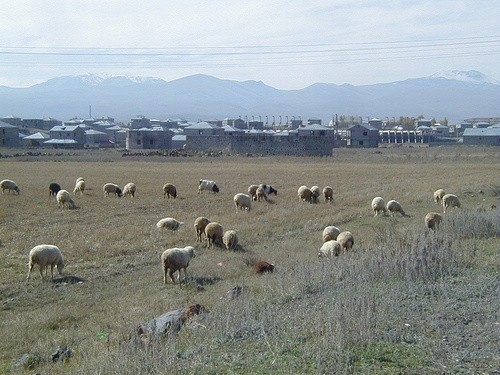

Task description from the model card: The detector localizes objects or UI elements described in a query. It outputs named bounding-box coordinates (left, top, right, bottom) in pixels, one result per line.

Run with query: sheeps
left=442, top=194, right=461, bottom=213
left=235, top=193, right=251, bottom=212
left=162, top=246, right=197, bottom=283
left=193, top=216, right=238, bottom=253
left=425, top=212, right=443, bottom=232
left=103, top=183, right=123, bottom=197
left=123, top=183, right=136, bottom=198
left=371, top=196, right=386, bottom=216
left=0, top=179, right=20, bottom=195
left=297, top=185, right=335, bottom=203
left=49, top=183, right=61, bottom=196
left=156, top=217, right=184, bottom=232
left=57, top=190, right=75, bottom=209
left=25, top=244, right=66, bottom=280
left=387, top=200, right=406, bottom=217
left=73, top=177, right=85, bottom=196
left=248, top=183, right=278, bottom=203
left=249, top=262, right=274, bottom=274
left=434, top=189, right=445, bottom=204
left=197, top=179, right=219, bottom=194
left=318, top=226, right=355, bottom=258
left=163, top=183, right=177, bottom=198
